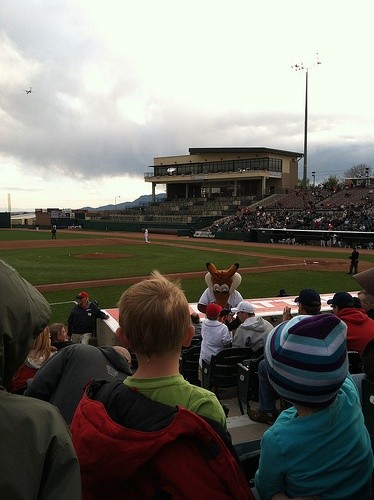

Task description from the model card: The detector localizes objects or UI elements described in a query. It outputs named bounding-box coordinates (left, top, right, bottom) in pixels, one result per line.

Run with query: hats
left=353, top=268, right=374, bottom=295
left=76, top=292, right=89, bottom=299
left=206, top=304, right=222, bottom=315
left=220, top=309, right=235, bottom=316
left=265, top=315, right=349, bottom=407
left=327, top=292, right=354, bottom=306
left=231, top=302, right=254, bottom=313
left=295, top=289, right=322, bottom=306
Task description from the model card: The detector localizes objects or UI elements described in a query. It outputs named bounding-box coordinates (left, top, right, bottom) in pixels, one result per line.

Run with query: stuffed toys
left=197, top=261, right=243, bottom=315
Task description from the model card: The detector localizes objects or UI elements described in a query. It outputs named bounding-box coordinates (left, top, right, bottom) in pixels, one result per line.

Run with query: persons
left=51, top=227, right=57, bottom=238
left=248, top=267, right=374, bottom=441
left=6, top=292, right=138, bottom=433
left=248, top=314, right=374, bottom=500
left=0, top=257, right=82, bottom=500
left=70, top=269, right=243, bottom=499
left=211, top=182, right=374, bottom=247
left=348, top=248, right=360, bottom=274
left=174, top=202, right=188, bottom=211
left=145, top=228, right=148, bottom=243
left=36, top=222, right=40, bottom=231
left=181, top=301, right=275, bottom=399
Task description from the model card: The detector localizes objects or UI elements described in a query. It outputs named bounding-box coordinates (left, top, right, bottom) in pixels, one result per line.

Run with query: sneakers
left=248, top=410, right=279, bottom=425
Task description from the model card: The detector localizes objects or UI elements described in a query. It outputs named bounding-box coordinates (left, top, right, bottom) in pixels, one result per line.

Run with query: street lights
left=311, top=171, right=316, bottom=188
left=114, top=195, right=120, bottom=215
left=291, top=61, right=309, bottom=184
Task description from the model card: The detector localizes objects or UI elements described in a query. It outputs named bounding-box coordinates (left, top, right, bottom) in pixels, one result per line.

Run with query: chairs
left=182, top=335, right=264, bottom=416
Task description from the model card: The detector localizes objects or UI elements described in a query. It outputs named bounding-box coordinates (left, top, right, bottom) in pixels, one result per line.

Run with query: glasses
left=297, top=302, right=300, bottom=307
left=330, top=305, right=337, bottom=308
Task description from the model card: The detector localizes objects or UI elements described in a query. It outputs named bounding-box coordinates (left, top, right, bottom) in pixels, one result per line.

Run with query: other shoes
left=346, top=272, right=351, bottom=274
